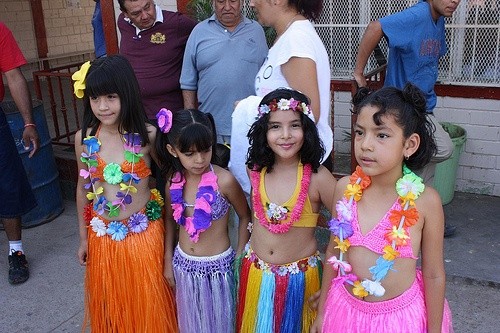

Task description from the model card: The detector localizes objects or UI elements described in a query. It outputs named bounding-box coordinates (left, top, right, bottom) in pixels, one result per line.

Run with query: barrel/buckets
left=0, top=100, right=65, bottom=230
left=424, top=122, right=466, bottom=206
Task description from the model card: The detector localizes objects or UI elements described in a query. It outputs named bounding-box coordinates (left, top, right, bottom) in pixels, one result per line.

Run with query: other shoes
left=443, top=223, right=457, bottom=238
left=8, top=249, right=29, bottom=284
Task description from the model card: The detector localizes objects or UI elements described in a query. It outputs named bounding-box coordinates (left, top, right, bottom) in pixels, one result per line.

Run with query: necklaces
left=250, top=159, right=312, bottom=234
left=168, top=164, right=218, bottom=244
left=80, top=118, right=142, bottom=217
left=325, top=163, right=425, bottom=297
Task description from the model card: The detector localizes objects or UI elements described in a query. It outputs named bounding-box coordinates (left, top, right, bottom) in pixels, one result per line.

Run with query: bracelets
left=23, top=123, right=37, bottom=128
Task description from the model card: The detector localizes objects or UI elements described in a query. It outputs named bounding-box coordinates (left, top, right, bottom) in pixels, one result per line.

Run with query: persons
left=151, top=108, right=253, bottom=333
left=90, top=0, right=108, bottom=58
left=311, top=81, right=456, bottom=333
left=71, top=54, right=179, bottom=333
left=0, top=22, right=41, bottom=285
left=117, top=0, right=198, bottom=206
left=231, top=0, right=334, bottom=241
left=178, top=0, right=271, bottom=171
left=369, top=1, right=499, bottom=83
left=229, top=87, right=338, bottom=333
left=353, top=0, right=459, bottom=238
